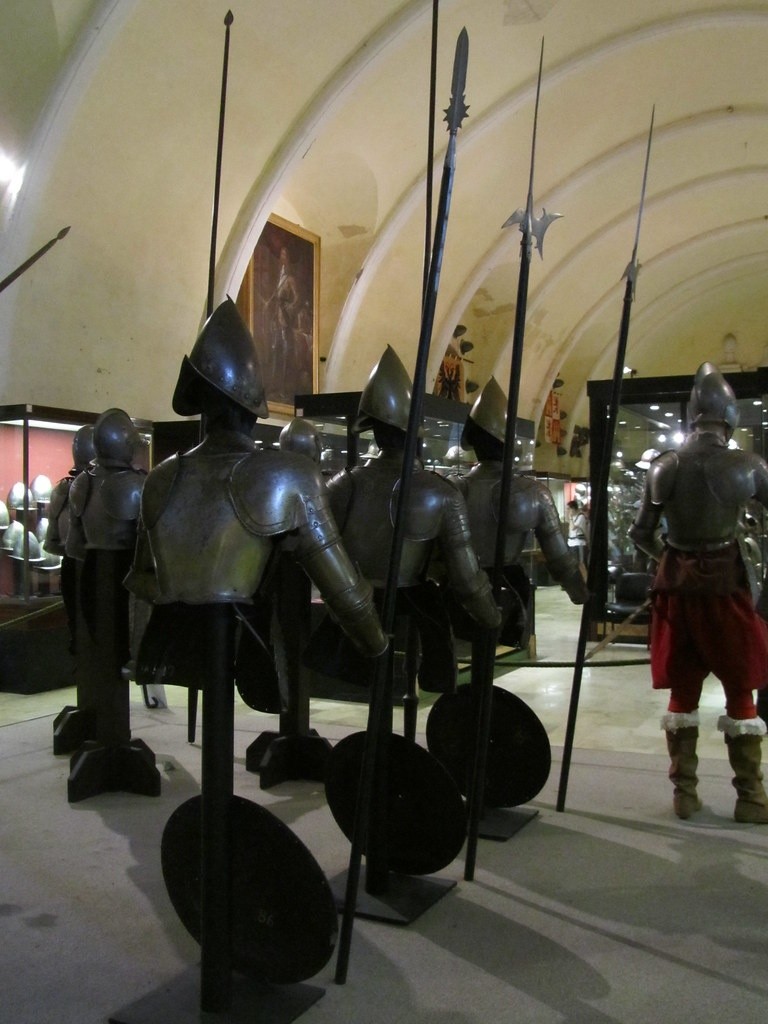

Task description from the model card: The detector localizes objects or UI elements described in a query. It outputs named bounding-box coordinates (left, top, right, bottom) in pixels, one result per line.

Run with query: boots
left=665, top=707, right=704, bottom=820
left=718, top=713, right=768, bottom=824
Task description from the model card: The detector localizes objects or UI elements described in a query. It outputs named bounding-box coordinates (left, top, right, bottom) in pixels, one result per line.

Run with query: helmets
left=88, top=407, right=151, bottom=474
left=687, top=362, right=739, bottom=441
left=279, top=417, right=321, bottom=463
left=0, top=475, right=68, bottom=571
left=459, top=376, right=516, bottom=451
left=172, top=296, right=270, bottom=419
left=321, top=442, right=466, bottom=474
left=353, top=344, right=425, bottom=440
left=68, top=424, right=96, bottom=476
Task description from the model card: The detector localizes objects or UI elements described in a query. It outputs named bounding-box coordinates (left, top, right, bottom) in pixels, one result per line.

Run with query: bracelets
left=569, top=519, right=573, bottom=520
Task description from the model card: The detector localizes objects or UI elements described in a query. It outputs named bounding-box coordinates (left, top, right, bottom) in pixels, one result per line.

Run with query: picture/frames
left=240, top=213, right=322, bottom=417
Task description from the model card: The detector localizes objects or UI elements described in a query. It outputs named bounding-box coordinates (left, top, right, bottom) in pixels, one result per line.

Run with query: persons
left=130, top=299, right=407, bottom=712
left=317, top=344, right=507, bottom=673
left=628, top=359, right=768, bottom=825
left=258, top=419, right=330, bottom=609
left=567, top=498, right=593, bottom=580
left=440, top=377, right=604, bottom=645
left=44, top=408, right=151, bottom=743
